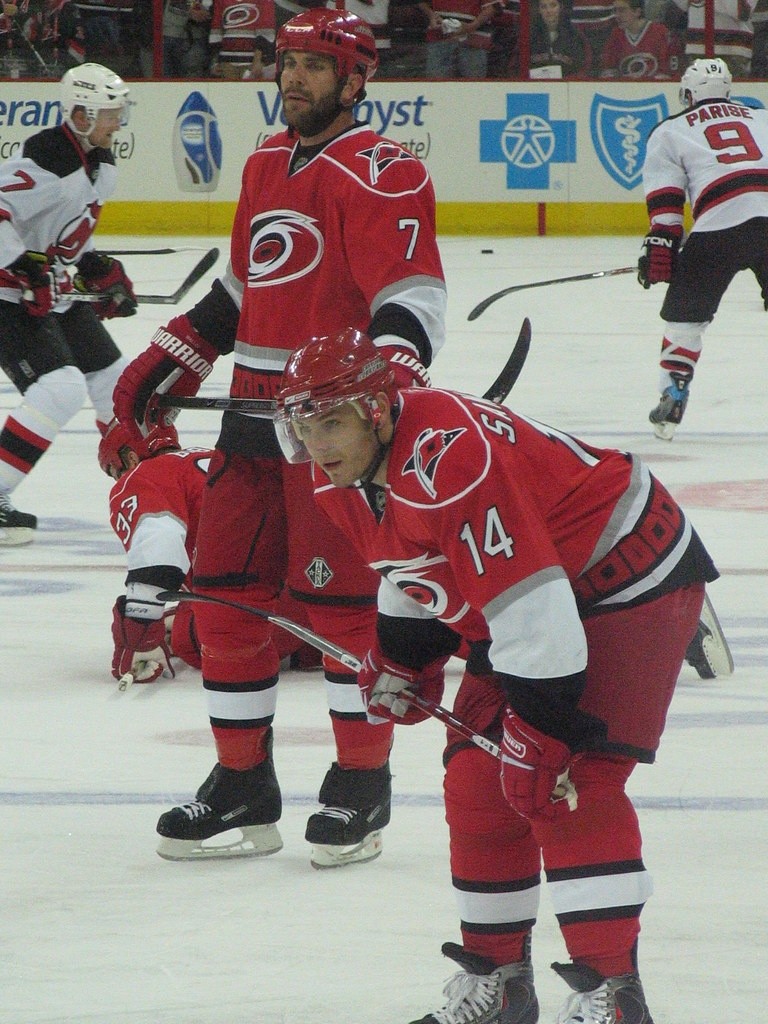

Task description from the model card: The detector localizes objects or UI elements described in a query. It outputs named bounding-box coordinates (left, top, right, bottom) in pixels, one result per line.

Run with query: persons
left=0, top=64, right=139, bottom=547
left=112, top=9, right=448, bottom=870
left=98, top=415, right=322, bottom=683
left=276, top=328, right=720, bottom=1024
left=0, top=0, right=391, bottom=78
left=416, top=0, right=759, bottom=78
left=636, top=58, right=768, bottom=441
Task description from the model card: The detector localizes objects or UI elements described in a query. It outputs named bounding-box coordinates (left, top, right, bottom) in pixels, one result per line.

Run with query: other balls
left=481, top=249, right=493, bottom=254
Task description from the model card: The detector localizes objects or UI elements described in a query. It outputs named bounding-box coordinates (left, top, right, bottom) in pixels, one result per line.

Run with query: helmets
left=98, top=414, right=181, bottom=480
left=58, top=63, right=130, bottom=135
left=272, top=325, right=400, bottom=427
left=680, top=58, right=733, bottom=106
left=274, top=6, right=379, bottom=82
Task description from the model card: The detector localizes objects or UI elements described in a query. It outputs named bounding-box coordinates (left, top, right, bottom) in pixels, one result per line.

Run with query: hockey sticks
left=159, top=317, right=532, bottom=414
left=24, top=247, right=220, bottom=305
left=117, top=632, right=172, bottom=693
left=155, top=590, right=565, bottom=797
left=92, top=246, right=211, bottom=255
left=467, top=266, right=640, bottom=321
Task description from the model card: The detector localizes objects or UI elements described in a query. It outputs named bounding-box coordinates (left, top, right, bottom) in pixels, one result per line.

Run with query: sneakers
left=155, top=726, right=282, bottom=860
left=408, top=932, right=538, bottom=1024
left=683, top=588, right=733, bottom=680
left=0, top=493, right=38, bottom=545
left=647, top=369, right=694, bottom=441
left=550, top=938, right=654, bottom=1024
left=304, top=762, right=392, bottom=869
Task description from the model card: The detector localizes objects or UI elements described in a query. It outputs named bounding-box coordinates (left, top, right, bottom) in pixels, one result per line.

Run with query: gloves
left=74, top=254, right=138, bottom=320
left=0, top=252, right=52, bottom=318
left=112, top=315, right=219, bottom=442
left=379, top=343, right=430, bottom=389
left=111, top=595, right=176, bottom=685
left=500, top=700, right=578, bottom=821
left=638, top=230, right=679, bottom=286
left=356, top=636, right=451, bottom=725
